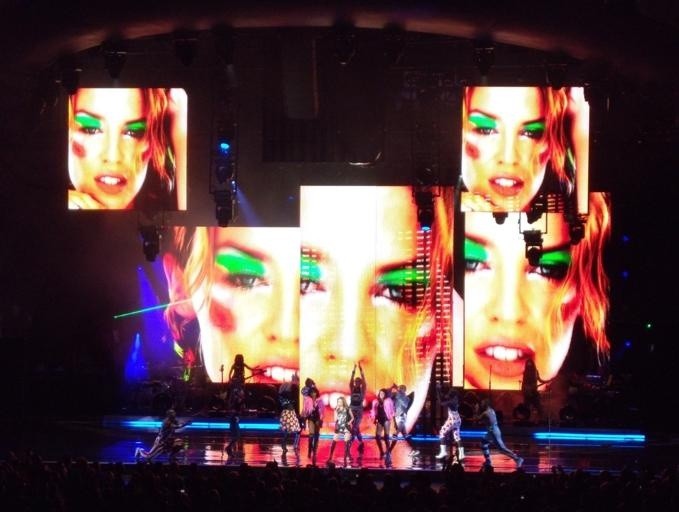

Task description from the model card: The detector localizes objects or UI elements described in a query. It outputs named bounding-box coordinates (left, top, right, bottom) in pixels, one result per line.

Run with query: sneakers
left=517, top=458, right=523, bottom=467
left=135, top=448, right=141, bottom=459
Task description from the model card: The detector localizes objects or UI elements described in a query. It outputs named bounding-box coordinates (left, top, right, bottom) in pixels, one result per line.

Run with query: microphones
left=220, top=365, right=224, bottom=372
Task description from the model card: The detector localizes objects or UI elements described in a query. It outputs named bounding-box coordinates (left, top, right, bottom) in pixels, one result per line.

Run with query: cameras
left=180, top=489, right=186, bottom=493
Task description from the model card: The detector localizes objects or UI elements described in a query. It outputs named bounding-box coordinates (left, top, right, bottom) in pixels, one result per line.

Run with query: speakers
left=608, top=444, right=679, bottom=474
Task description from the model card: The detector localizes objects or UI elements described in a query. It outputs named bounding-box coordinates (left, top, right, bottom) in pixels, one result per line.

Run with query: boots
left=225, top=439, right=237, bottom=456
left=280, top=434, right=400, bottom=467
left=457, top=447, right=465, bottom=460
left=435, top=445, right=448, bottom=459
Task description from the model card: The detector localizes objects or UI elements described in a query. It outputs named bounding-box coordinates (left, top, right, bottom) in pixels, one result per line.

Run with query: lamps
left=210, top=25, right=242, bottom=226
left=568, top=218, right=584, bottom=245
left=138, top=225, right=164, bottom=263
left=411, top=159, right=442, bottom=232
left=50, top=30, right=201, bottom=91
left=523, top=229, right=543, bottom=267
left=333, top=23, right=358, bottom=67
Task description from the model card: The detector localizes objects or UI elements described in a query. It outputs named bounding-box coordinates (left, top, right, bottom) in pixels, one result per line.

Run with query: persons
left=464, top=193, right=613, bottom=391
left=161, top=226, right=300, bottom=385
left=461, top=86, right=589, bottom=214
left=300, top=186, right=454, bottom=435
left=68, top=87, right=187, bottom=211
